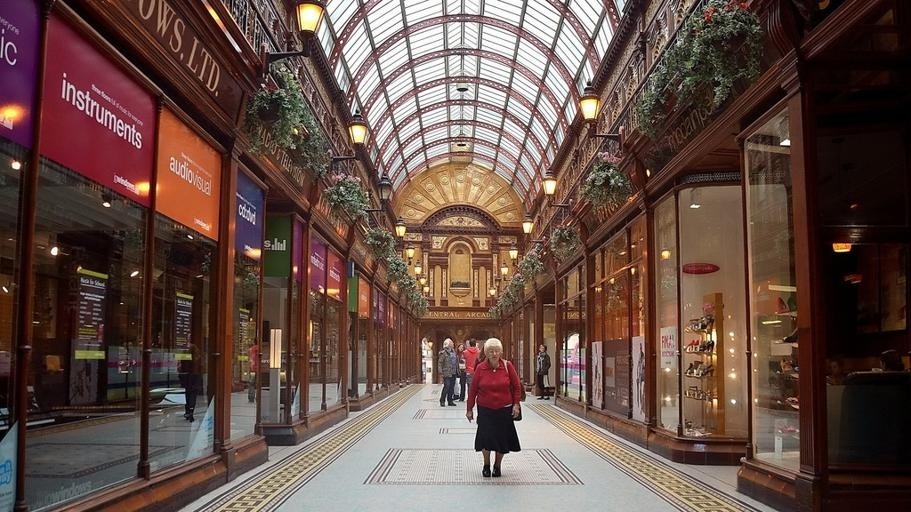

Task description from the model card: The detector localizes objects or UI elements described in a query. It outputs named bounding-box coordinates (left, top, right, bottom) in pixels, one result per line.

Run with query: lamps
left=578, top=80, right=627, bottom=153
left=326, top=110, right=432, bottom=299
left=260, top=0, right=329, bottom=76
left=488, top=171, right=578, bottom=301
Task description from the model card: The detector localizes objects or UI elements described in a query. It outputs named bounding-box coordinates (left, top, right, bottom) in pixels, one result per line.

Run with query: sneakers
left=683, top=318, right=714, bottom=378
left=777, top=295, right=799, bottom=343
left=441, top=395, right=464, bottom=407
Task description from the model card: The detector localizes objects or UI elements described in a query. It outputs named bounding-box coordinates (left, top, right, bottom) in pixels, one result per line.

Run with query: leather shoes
left=492, top=464, right=501, bottom=477
left=483, top=464, right=491, bottom=477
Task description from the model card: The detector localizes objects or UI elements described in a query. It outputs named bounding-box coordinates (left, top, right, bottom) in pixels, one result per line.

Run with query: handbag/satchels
left=543, top=374, right=550, bottom=388
left=512, top=402, right=522, bottom=421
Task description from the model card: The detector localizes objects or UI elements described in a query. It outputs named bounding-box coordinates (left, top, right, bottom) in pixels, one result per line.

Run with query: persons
left=437, top=338, right=481, bottom=407
left=466, top=338, right=522, bottom=477
left=248, top=336, right=258, bottom=402
left=536, top=344, right=551, bottom=400
left=180, top=343, right=204, bottom=421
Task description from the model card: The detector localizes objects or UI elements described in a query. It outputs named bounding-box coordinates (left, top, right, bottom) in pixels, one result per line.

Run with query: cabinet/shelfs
left=684, top=316, right=720, bottom=400
